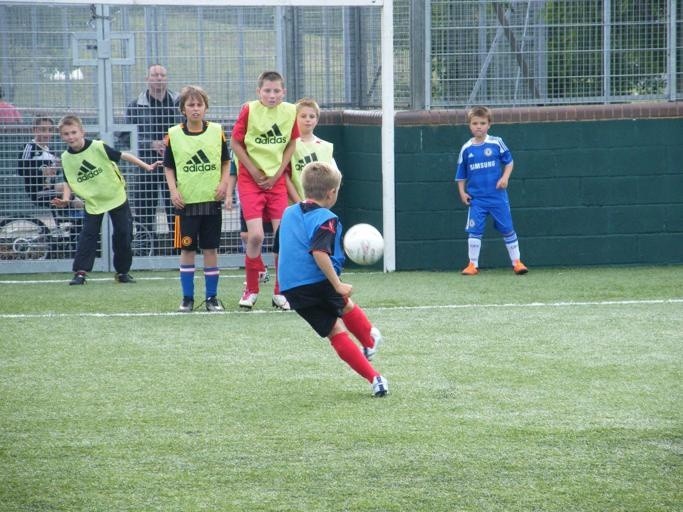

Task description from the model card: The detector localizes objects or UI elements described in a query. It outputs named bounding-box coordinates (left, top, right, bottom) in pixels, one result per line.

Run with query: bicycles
left=0, top=167, right=154, bottom=257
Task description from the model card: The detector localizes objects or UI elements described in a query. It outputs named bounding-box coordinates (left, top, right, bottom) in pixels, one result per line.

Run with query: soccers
left=342, top=223, right=384, bottom=267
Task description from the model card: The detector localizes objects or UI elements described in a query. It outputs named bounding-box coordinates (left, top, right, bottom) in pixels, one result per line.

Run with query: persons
left=455, top=107, right=528, bottom=274
left=124, top=64, right=186, bottom=256
left=163, top=84, right=230, bottom=310
left=50, top=115, right=164, bottom=285
left=230, top=72, right=301, bottom=311
left=1, top=88, right=20, bottom=132
left=284, top=98, right=334, bottom=207
left=223, top=146, right=270, bottom=283
left=18, top=112, right=85, bottom=258
left=272, top=163, right=390, bottom=396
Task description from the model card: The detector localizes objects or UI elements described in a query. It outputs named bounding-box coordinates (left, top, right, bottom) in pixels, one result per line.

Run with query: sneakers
left=270, top=294, right=293, bottom=310
left=361, top=326, right=381, bottom=360
left=459, top=263, right=479, bottom=275
left=114, top=271, right=136, bottom=283
left=511, top=260, right=530, bottom=276
left=68, top=272, right=87, bottom=285
left=204, top=294, right=226, bottom=313
left=238, top=289, right=258, bottom=310
left=369, top=373, right=390, bottom=398
left=243, top=268, right=269, bottom=285
left=179, top=296, right=195, bottom=313
